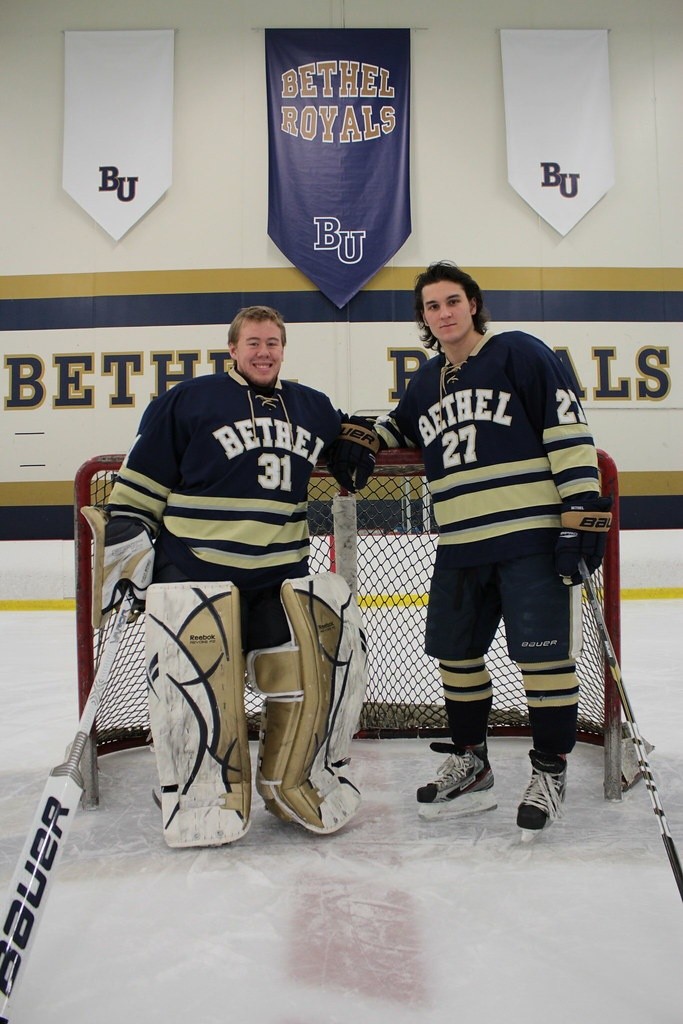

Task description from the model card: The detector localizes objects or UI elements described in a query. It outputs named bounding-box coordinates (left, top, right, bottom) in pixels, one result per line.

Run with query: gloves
left=327, top=414, right=382, bottom=493
left=554, top=498, right=612, bottom=588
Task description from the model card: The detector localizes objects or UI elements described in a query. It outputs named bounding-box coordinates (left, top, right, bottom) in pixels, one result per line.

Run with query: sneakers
left=416, top=733, right=498, bottom=821
left=515, top=747, right=567, bottom=844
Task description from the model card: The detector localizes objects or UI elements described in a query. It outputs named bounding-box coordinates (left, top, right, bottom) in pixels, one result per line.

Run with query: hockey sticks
left=578, top=557, right=683, bottom=904
left=0, top=585, right=135, bottom=1024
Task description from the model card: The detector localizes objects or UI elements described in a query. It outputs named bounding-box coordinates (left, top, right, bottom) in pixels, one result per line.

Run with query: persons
left=82, top=306, right=369, bottom=850
left=328, top=265, right=613, bottom=841
